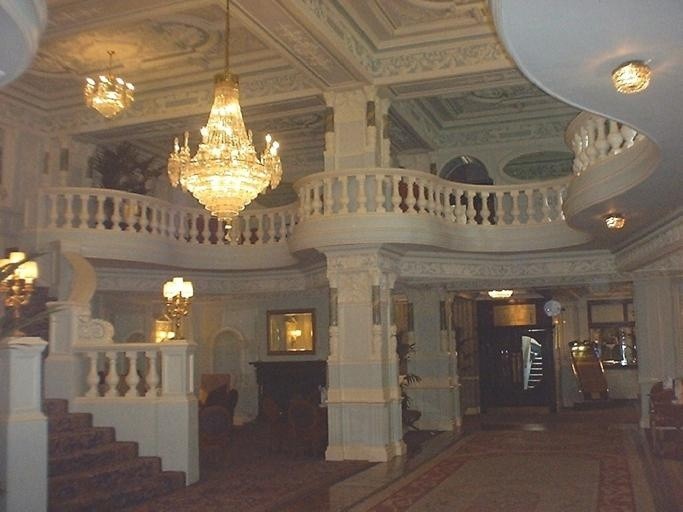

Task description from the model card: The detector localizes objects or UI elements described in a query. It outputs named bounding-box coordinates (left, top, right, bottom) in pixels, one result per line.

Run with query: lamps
left=0, top=250, right=40, bottom=336
left=611, top=59, right=651, bottom=95
left=83, top=50, right=134, bottom=122
left=166, top=2, right=283, bottom=225
left=602, top=214, right=627, bottom=232
left=161, top=276, right=194, bottom=340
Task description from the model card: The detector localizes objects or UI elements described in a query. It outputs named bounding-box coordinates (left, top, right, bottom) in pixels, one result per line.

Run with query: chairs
left=200, top=372, right=327, bottom=453
left=647, top=379, right=682, bottom=456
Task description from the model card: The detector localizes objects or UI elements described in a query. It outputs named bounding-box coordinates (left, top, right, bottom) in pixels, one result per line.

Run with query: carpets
left=336, top=417, right=682, bottom=512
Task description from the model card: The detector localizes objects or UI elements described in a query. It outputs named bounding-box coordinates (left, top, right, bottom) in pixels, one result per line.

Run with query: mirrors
left=265, top=306, right=318, bottom=354
